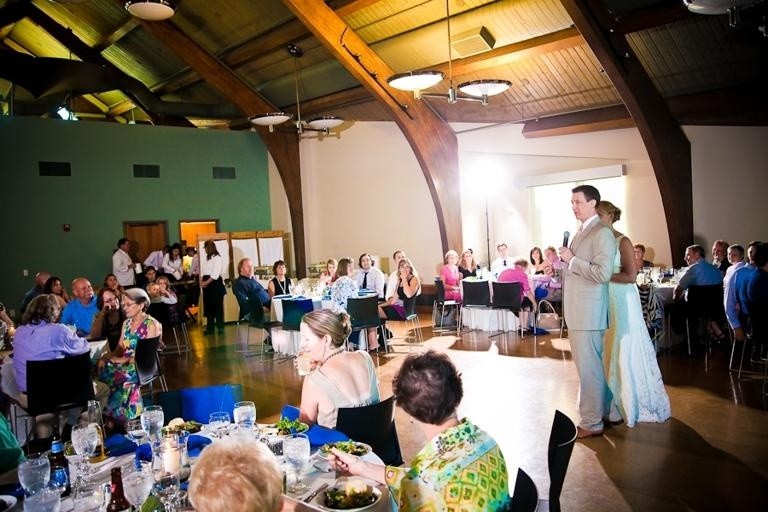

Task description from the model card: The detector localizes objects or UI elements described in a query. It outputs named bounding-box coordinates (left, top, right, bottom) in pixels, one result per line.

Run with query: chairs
left=235, top=278, right=423, bottom=372
left=432, top=275, right=565, bottom=336
left=337, top=395, right=404, bottom=467
left=637, top=274, right=768, bottom=380
left=2, top=291, right=190, bottom=454
left=509, top=410, right=579, bottom=512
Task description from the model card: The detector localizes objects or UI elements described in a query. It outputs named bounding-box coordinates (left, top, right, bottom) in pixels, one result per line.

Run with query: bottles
left=86, top=400, right=109, bottom=454
left=50, top=427, right=71, bottom=497
left=1, top=324, right=15, bottom=349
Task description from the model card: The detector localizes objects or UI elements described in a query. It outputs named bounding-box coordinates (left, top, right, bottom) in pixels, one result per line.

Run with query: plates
left=318, top=442, right=372, bottom=461
left=0, top=494, right=18, bottom=511
left=262, top=421, right=309, bottom=436
left=314, top=483, right=383, bottom=512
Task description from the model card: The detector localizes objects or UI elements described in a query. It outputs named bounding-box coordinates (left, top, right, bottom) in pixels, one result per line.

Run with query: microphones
left=561, top=231, right=570, bottom=262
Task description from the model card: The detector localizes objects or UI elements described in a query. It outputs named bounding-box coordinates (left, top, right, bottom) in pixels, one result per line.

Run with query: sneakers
left=203, top=326, right=225, bottom=334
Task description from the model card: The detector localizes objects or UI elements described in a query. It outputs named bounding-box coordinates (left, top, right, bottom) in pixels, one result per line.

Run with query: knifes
left=304, top=482, right=329, bottom=503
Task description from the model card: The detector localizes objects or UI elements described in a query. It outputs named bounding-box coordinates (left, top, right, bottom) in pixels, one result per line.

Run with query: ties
left=577, top=224, right=583, bottom=238
left=503, top=260, right=506, bottom=266
left=362, top=272, right=368, bottom=288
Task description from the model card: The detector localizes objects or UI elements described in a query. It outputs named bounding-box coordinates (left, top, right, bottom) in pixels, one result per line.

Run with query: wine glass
left=70, top=405, right=190, bottom=511
left=636, top=266, right=680, bottom=289
left=288, top=277, right=331, bottom=300
left=473, top=266, right=499, bottom=279
left=283, top=432, right=311, bottom=495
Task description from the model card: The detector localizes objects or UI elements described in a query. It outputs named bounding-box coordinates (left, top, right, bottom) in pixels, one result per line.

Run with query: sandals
left=520, top=326, right=533, bottom=334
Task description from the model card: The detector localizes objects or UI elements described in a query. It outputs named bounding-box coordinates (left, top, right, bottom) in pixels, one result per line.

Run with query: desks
left=5, top=419, right=389, bottom=511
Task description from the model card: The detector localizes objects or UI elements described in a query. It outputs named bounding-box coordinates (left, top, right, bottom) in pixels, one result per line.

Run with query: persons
left=559, top=185, right=624, bottom=439
left=596, top=202, right=671, bottom=428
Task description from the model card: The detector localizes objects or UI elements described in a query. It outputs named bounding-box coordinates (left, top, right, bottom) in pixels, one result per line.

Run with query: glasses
left=104, top=297, right=119, bottom=303
left=127, top=301, right=136, bottom=307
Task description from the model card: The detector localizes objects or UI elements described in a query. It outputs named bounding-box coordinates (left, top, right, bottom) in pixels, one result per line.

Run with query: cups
left=66, top=323, right=76, bottom=334
left=298, top=352, right=310, bottom=376
left=209, top=400, right=256, bottom=437
left=17, top=452, right=61, bottom=511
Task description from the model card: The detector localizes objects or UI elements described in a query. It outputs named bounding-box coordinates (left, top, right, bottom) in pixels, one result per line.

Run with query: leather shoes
left=575, top=426, right=603, bottom=439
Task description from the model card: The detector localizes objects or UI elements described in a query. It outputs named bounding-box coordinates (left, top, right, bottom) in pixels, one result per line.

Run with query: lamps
left=681, top=1, right=760, bottom=29
left=385, top=1, right=513, bottom=108
left=123, top=0, right=178, bottom=21
left=247, top=44, right=346, bottom=136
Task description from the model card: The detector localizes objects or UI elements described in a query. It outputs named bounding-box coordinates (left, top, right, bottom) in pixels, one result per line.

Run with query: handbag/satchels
left=433, top=306, right=456, bottom=326
left=535, top=300, right=560, bottom=331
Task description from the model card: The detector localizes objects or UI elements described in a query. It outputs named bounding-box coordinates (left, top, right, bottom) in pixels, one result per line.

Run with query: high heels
left=365, top=345, right=381, bottom=354
